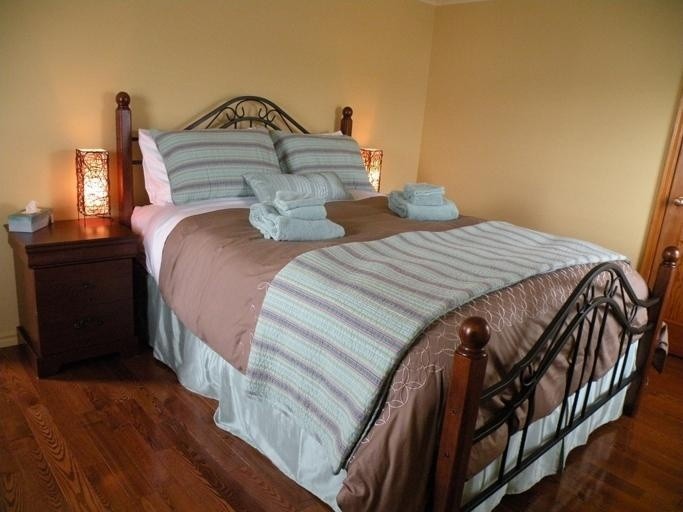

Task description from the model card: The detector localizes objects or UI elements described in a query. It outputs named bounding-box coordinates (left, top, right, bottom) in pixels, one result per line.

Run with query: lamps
left=358, top=148, right=382, bottom=193
left=75, top=148, right=110, bottom=217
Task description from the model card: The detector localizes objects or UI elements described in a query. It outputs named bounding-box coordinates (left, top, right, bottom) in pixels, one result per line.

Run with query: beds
left=115, top=92, right=679, bottom=512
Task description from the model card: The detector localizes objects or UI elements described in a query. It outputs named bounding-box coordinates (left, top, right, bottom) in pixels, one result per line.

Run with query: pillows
left=151, top=129, right=281, bottom=205
left=136, top=127, right=257, bottom=204
left=269, top=129, right=374, bottom=192
left=239, top=171, right=355, bottom=202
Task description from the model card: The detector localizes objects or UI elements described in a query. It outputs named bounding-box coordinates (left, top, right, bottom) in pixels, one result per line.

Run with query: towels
left=273, top=190, right=328, bottom=220
left=388, top=191, right=459, bottom=220
left=404, top=182, right=446, bottom=206
left=250, top=205, right=345, bottom=240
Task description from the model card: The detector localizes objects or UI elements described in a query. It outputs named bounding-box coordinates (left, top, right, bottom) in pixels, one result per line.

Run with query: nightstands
left=3, top=217, right=139, bottom=379
